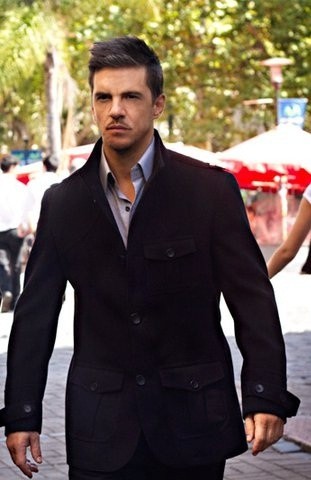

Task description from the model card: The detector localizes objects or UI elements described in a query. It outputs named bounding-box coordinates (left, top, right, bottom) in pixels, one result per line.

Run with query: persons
left=0, top=38, right=302, bottom=480
left=0, top=154, right=311, bottom=313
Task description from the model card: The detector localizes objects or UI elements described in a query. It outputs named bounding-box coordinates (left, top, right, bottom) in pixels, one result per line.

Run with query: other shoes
left=10, top=304, right=15, bottom=310
left=0, top=291, right=12, bottom=312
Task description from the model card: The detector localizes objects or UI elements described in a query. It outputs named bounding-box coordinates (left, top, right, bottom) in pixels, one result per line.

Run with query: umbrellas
left=220, top=122, right=311, bottom=240
